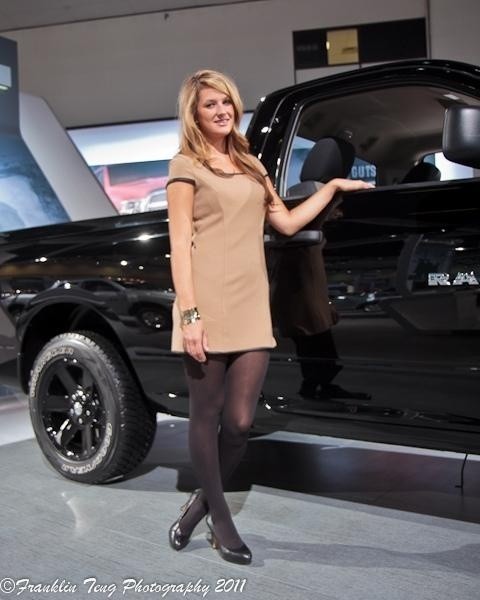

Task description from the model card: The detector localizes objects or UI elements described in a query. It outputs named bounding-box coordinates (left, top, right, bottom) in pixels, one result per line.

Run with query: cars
left=1, top=276, right=177, bottom=333
left=327, top=282, right=392, bottom=315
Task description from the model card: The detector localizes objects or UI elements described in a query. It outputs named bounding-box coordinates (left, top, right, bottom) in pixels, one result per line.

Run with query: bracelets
left=180, top=307, right=202, bottom=328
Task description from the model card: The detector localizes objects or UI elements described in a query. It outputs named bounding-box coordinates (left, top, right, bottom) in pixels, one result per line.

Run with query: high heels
left=203, top=513, right=253, bottom=568
left=167, top=488, right=207, bottom=554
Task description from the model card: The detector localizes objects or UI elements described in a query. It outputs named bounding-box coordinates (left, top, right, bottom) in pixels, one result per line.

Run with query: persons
left=164, top=65, right=374, bottom=566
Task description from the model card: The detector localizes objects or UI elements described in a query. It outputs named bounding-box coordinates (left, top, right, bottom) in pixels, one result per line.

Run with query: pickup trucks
left=0, top=56, right=479, bottom=484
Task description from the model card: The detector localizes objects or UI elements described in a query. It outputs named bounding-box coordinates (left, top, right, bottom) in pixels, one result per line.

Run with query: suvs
left=93, top=159, right=169, bottom=216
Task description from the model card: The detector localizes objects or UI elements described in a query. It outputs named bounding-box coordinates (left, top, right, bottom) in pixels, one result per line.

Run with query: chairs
left=288, top=135, right=442, bottom=203
